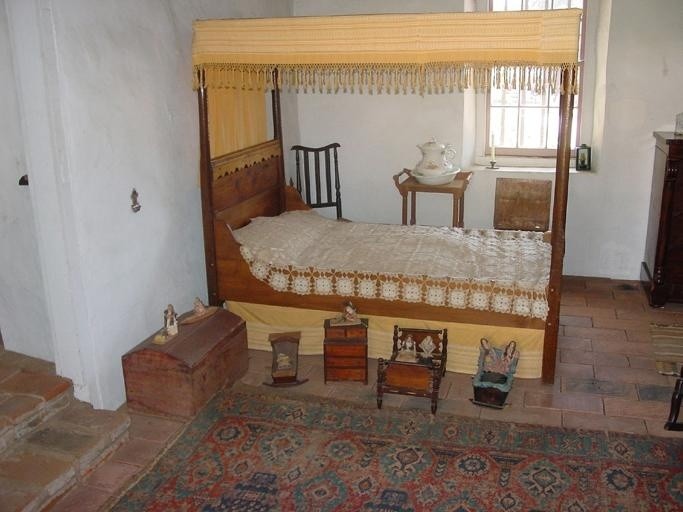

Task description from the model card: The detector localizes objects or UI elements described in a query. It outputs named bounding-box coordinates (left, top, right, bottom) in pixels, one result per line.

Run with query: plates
left=410, top=167, right=461, bottom=186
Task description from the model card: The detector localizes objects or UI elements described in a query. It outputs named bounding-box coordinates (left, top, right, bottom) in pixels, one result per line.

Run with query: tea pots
left=415, top=136, right=456, bottom=176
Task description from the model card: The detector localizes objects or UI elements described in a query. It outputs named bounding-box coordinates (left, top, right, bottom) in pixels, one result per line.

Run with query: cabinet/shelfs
left=640, top=131, right=683, bottom=309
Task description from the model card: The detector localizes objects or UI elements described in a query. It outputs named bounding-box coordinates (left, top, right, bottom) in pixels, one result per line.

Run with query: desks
left=393, top=169, right=470, bottom=229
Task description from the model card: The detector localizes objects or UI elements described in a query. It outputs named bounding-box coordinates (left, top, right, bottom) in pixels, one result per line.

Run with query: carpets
left=95, top=389, right=683, bottom=511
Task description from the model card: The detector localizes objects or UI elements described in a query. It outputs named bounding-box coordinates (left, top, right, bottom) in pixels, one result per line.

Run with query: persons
left=162, top=298, right=207, bottom=335
left=395, top=334, right=436, bottom=365
left=480, top=338, right=516, bottom=374
left=329, top=301, right=362, bottom=327
left=580, top=154, right=585, bottom=167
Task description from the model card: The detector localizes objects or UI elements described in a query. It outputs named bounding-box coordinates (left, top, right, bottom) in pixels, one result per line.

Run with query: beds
left=193, top=4, right=585, bottom=388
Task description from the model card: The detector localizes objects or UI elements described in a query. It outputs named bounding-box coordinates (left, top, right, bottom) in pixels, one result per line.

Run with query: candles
left=490, top=132, right=497, bottom=162
